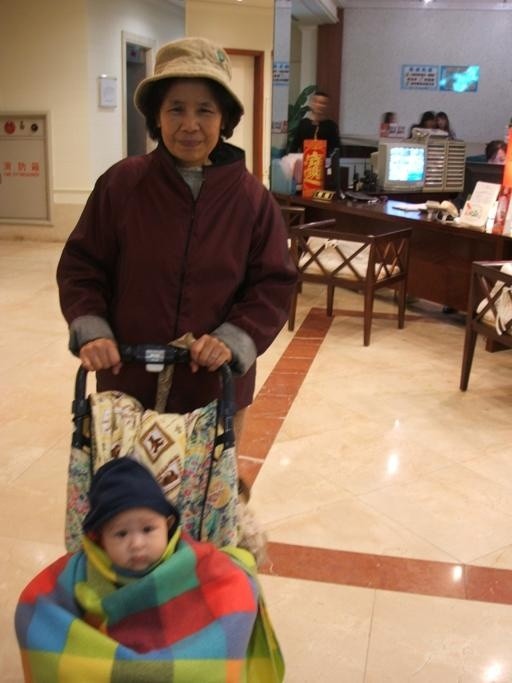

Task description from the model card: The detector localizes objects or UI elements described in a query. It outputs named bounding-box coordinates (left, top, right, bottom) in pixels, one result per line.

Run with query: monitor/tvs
left=371, top=141, right=427, bottom=193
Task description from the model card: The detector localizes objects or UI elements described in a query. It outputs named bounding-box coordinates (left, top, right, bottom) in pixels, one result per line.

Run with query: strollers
left=64, top=340, right=236, bottom=681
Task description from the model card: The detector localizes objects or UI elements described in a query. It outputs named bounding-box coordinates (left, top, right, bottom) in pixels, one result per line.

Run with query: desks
left=270, top=191, right=512, bottom=312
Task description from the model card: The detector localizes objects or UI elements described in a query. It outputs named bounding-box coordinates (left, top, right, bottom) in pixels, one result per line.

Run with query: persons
left=57, top=37, right=301, bottom=459
left=380, top=112, right=395, bottom=137
left=442, top=140, right=507, bottom=315
left=290, top=92, right=340, bottom=156
left=436, top=112, right=454, bottom=140
left=16, top=457, right=285, bottom=683
left=408, top=111, right=436, bottom=138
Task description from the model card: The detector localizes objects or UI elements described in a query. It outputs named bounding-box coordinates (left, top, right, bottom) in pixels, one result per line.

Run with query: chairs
left=291, top=219, right=412, bottom=347
left=279, top=207, right=305, bottom=266
left=460, top=260, right=512, bottom=389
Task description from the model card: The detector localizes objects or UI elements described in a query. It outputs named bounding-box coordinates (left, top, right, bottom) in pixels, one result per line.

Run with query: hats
left=81, top=455, right=180, bottom=539
left=134, top=37, right=245, bottom=128
